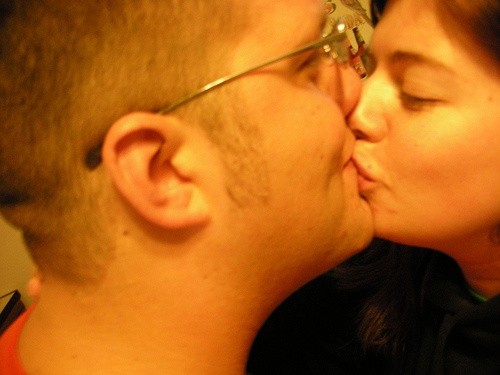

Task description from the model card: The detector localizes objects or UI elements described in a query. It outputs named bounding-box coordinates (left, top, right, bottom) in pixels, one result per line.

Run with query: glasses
left=82, top=11, right=360, bottom=168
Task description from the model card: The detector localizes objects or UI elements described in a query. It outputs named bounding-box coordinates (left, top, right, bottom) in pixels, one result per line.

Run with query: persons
left=346, top=0, right=500, bottom=374
left=1, top=0, right=374, bottom=374
